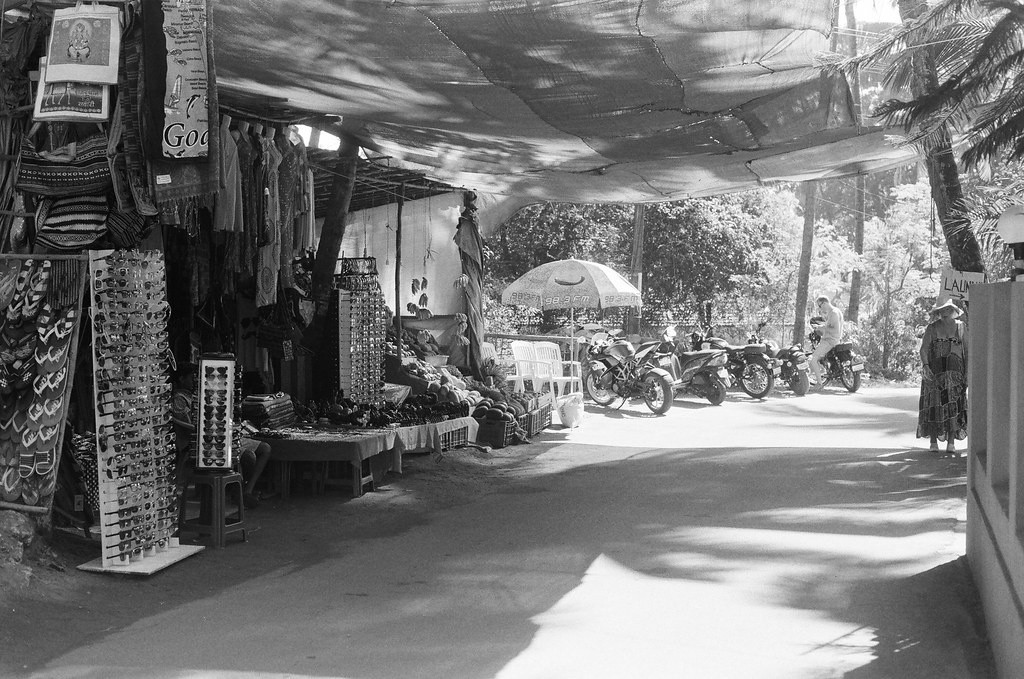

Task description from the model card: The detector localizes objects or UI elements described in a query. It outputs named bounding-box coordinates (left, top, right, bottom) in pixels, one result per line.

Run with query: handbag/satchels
left=256, top=270, right=305, bottom=353
left=14, top=0, right=158, bottom=250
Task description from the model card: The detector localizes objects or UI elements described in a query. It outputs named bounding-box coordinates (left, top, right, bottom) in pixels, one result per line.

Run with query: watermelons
left=428, top=374, right=516, bottom=421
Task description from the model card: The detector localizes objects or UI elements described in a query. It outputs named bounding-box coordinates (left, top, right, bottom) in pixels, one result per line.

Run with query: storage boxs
left=385, top=316, right=553, bottom=455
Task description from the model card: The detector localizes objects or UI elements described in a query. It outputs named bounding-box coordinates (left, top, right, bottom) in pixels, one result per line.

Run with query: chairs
left=184, top=470, right=247, bottom=545
left=482, top=338, right=583, bottom=400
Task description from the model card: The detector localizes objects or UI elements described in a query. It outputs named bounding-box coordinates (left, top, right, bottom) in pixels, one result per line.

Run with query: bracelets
left=923, top=364, right=929, bottom=367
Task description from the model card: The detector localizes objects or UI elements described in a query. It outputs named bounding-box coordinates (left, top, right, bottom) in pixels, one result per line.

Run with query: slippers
left=0, top=257, right=80, bottom=506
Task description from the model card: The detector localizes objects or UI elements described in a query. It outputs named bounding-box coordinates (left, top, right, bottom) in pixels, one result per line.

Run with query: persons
left=916, top=294, right=968, bottom=452
left=809, top=297, right=843, bottom=390
left=213, top=115, right=316, bottom=276
left=174, top=361, right=272, bottom=504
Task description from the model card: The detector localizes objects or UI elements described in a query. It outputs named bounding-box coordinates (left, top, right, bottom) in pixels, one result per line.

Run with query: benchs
left=269, top=431, right=393, bottom=503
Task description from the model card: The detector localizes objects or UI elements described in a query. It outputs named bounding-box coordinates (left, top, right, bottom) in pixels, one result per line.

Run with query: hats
left=928, top=301, right=964, bottom=319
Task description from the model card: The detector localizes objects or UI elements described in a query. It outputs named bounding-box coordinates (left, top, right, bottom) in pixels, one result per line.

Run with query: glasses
left=341, top=290, right=387, bottom=407
left=93, top=255, right=180, bottom=561
left=202, top=367, right=228, bottom=466
left=819, top=302, right=823, bottom=308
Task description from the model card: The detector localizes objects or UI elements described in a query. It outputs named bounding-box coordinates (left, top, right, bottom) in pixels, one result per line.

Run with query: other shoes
left=929, top=444, right=939, bottom=452
left=814, top=382, right=823, bottom=392
left=946, top=444, right=955, bottom=453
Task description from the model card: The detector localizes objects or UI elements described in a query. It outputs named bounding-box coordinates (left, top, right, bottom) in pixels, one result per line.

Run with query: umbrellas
left=501, top=256, right=642, bottom=394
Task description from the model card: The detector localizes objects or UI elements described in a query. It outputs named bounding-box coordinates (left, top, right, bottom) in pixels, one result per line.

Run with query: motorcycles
left=800, top=317, right=864, bottom=392
left=577, top=329, right=674, bottom=415
left=743, top=319, right=812, bottom=398
left=676, top=327, right=783, bottom=399
left=648, top=328, right=731, bottom=406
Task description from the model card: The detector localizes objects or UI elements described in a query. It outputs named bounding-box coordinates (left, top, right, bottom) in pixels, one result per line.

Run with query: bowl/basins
left=425, top=355, right=449, bottom=366
